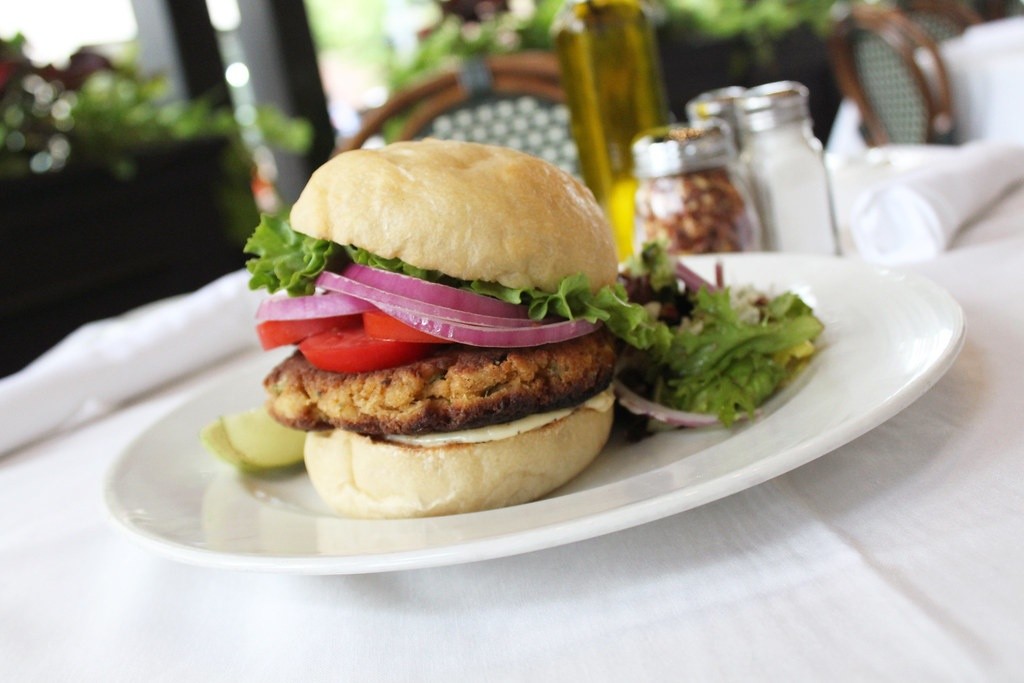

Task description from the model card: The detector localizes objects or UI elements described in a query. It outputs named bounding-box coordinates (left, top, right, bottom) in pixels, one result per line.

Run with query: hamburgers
left=244, top=139, right=676, bottom=519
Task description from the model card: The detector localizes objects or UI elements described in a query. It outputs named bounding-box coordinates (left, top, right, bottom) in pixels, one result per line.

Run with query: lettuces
left=611, top=238, right=824, bottom=434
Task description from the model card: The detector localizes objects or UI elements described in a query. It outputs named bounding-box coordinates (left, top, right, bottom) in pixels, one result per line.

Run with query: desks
left=828, top=18, right=1024, bottom=149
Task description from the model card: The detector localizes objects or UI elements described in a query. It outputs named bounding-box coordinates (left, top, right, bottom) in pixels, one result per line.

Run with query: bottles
left=628, top=79, right=840, bottom=260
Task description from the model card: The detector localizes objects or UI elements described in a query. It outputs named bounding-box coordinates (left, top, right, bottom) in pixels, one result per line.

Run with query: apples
left=198, top=411, right=304, bottom=469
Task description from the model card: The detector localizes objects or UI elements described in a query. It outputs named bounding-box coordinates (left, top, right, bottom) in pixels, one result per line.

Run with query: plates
left=92, top=250, right=970, bottom=574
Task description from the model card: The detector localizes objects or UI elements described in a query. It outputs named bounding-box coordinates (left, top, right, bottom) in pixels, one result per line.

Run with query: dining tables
left=0, top=151, right=1024, bottom=683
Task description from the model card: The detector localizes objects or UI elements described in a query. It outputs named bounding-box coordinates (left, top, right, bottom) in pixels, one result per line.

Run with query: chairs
left=823, top=0, right=984, bottom=147
left=329, top=51, right=584, bottom=187
